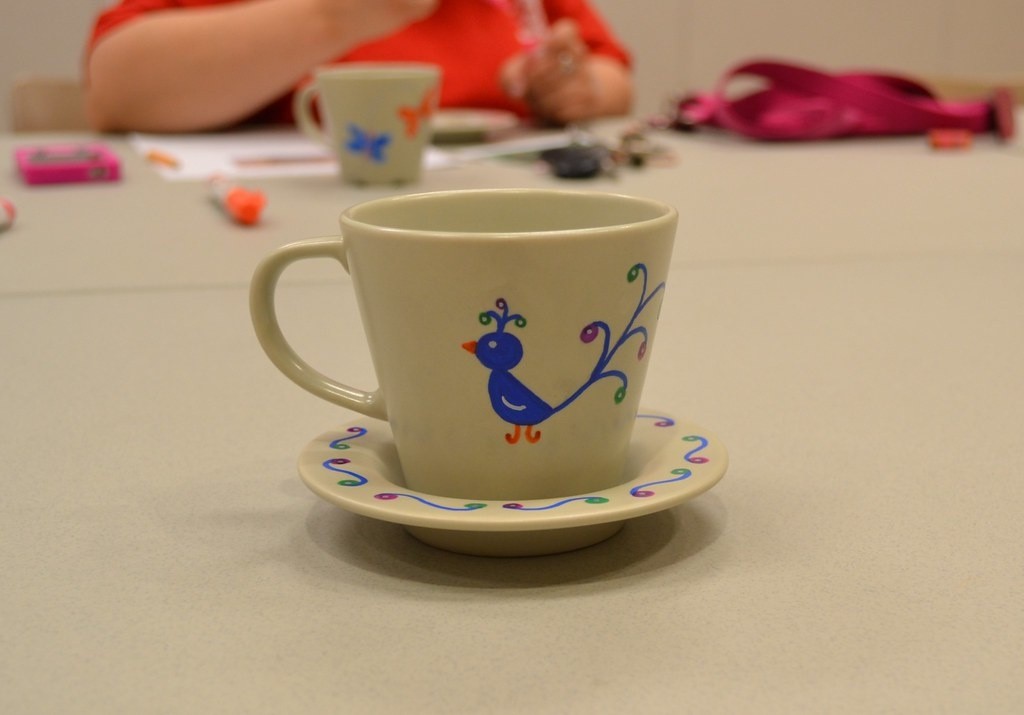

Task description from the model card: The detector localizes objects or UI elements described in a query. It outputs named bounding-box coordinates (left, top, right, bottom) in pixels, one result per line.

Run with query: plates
left=297, top=408, right=727, bottom=559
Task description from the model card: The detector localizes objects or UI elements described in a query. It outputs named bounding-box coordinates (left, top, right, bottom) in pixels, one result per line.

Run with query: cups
left=249, top=189, right=678, bottom=500
left=297, top=67, right=442, bottom=185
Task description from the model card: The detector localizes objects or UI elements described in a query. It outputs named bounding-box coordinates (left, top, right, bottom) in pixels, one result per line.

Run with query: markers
left=199, top=171, right=269, bottom=227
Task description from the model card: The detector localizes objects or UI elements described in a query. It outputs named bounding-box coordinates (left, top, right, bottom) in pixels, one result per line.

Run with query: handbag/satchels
left=678, top=59, right=990, bottom=140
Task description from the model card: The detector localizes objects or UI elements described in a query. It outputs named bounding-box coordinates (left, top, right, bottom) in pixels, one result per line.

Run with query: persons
left=77, top=1, right=639, bottom=136
